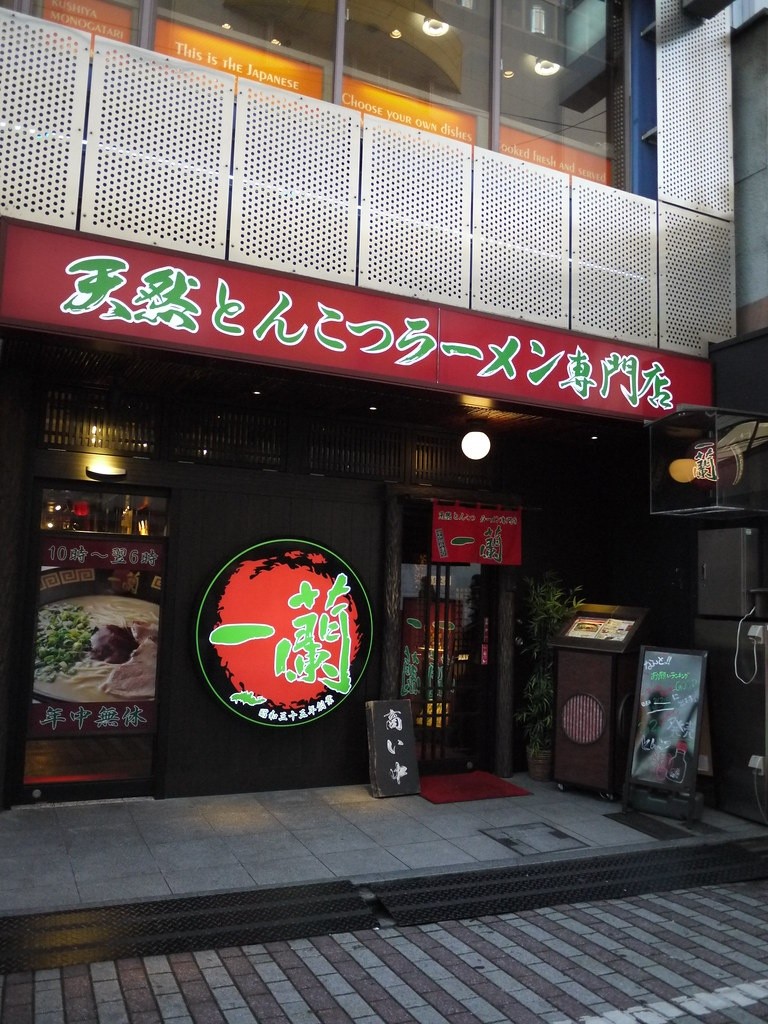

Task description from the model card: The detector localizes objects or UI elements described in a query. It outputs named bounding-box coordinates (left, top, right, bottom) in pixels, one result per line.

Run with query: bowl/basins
left=31, top=565, right=159, bottom=704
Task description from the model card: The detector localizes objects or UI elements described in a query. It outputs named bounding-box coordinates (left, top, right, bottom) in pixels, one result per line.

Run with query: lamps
left=450, top=421, right=494, bottom=463
left=535, top=56, right=560, bottom=77
left=421, top=15, right=450, bottom=37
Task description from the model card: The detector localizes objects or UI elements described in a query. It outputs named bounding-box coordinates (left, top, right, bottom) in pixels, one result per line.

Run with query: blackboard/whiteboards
left=627, top=647, right=710, bottom=797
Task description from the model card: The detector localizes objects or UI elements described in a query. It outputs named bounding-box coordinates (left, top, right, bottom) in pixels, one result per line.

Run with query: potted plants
left=511, top=572, right=587, bottom=782
